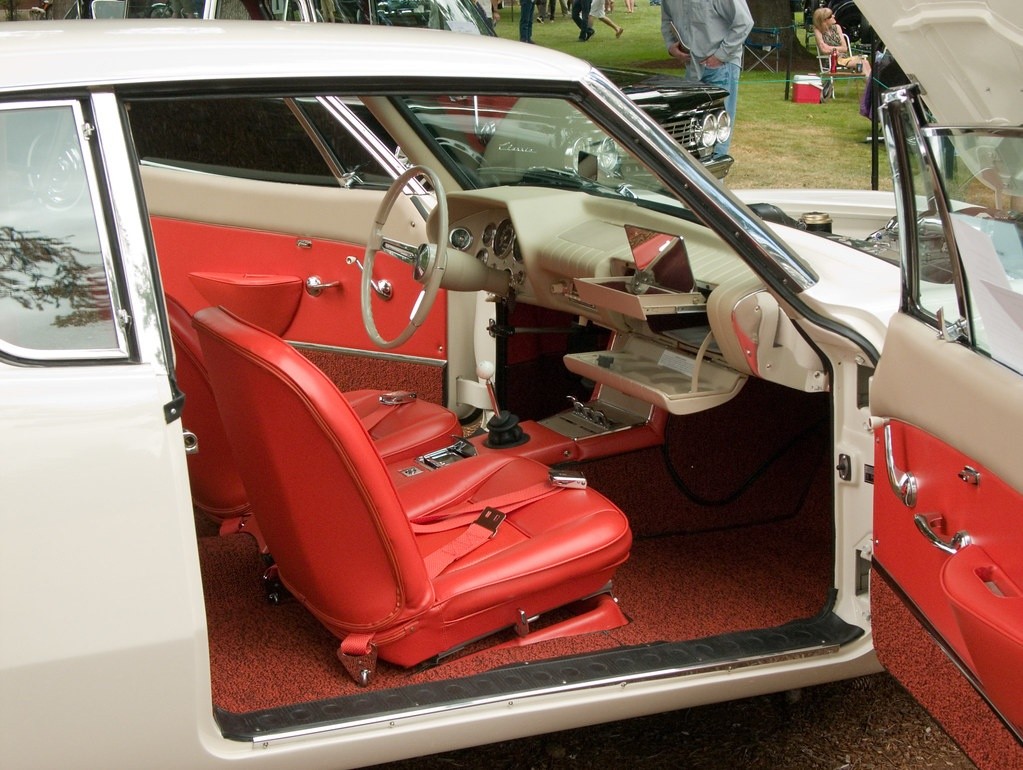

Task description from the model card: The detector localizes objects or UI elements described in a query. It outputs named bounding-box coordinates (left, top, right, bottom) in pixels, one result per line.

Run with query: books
left=670, top=21, right=690, bottom=66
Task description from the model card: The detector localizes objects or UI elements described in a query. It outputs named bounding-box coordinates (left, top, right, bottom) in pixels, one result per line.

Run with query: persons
left=813, top=8, right=872, bottom=80
left=475, top=0, right=635, bottom=44
left=661, top=0, right=754, bottom=182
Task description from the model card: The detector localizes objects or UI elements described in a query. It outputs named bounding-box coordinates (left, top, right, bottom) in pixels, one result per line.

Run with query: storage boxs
left=793, top=75, right=823, bottom=104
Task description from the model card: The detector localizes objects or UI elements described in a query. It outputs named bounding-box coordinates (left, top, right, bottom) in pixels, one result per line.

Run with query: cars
left=23, top=0, right=734, bottom=184
left=281, top=0, right=497, bottom=35
left=1, top=1, right=1021, bottom=770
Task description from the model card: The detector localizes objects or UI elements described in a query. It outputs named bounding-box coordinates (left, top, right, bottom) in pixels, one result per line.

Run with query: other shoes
left=549, top=20, right=555, bottom=24
left=576, top=37, right=586, bottom=42
left=615, top=28, right=624, bottom=39
left=528, top=40, right=536, bottom=44
left=536, top=16, right=544, bottom=24
left=586, top=31, right=596, bottom=42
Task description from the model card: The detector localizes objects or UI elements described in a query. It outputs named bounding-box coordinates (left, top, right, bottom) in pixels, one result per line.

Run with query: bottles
left=856, top=54, right=863, bottom=73
left=831, top=48, right=837, bottom=73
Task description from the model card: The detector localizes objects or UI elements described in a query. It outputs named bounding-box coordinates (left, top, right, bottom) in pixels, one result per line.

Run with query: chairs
left=90, top=250, right=464, bottom=553
left=190, top=303, right=635, bottom=683
left=741, top=26, right=784, bottom=73
left=815, top=34, right=868, bottom=99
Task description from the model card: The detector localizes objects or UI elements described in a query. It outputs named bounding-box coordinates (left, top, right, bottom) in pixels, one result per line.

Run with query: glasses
left=825, top=13, right=834, bottom=21
